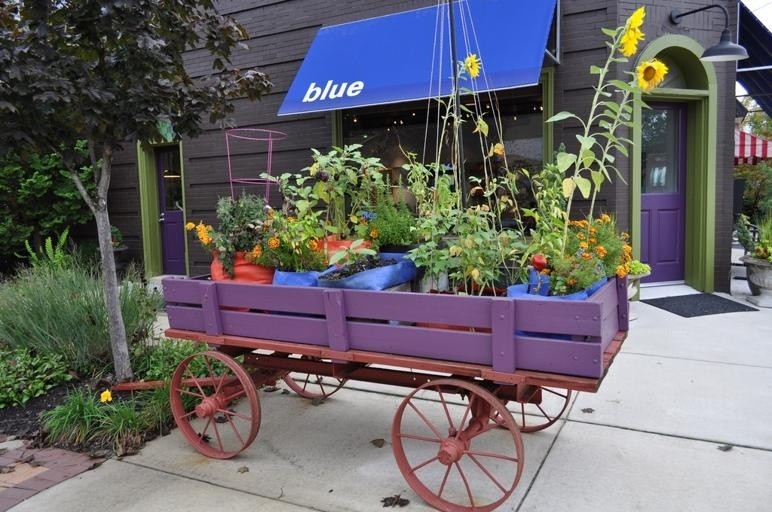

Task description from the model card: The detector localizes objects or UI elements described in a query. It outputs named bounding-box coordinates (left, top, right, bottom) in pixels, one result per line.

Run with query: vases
left=203, top=250, right=320, bottom=321
left=416, top=273, right=611, bottom=329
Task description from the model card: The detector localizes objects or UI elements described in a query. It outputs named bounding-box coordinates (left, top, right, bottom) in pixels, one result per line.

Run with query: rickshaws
left=108, top=248, right=630, bottom=512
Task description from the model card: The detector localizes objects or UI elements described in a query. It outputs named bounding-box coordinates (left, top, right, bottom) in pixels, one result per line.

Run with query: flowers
left=185, top=196, right=328, bottom=281
left=736, top=209, right=771, bottom=260
left=409, top=4, right=693, bottom=293
